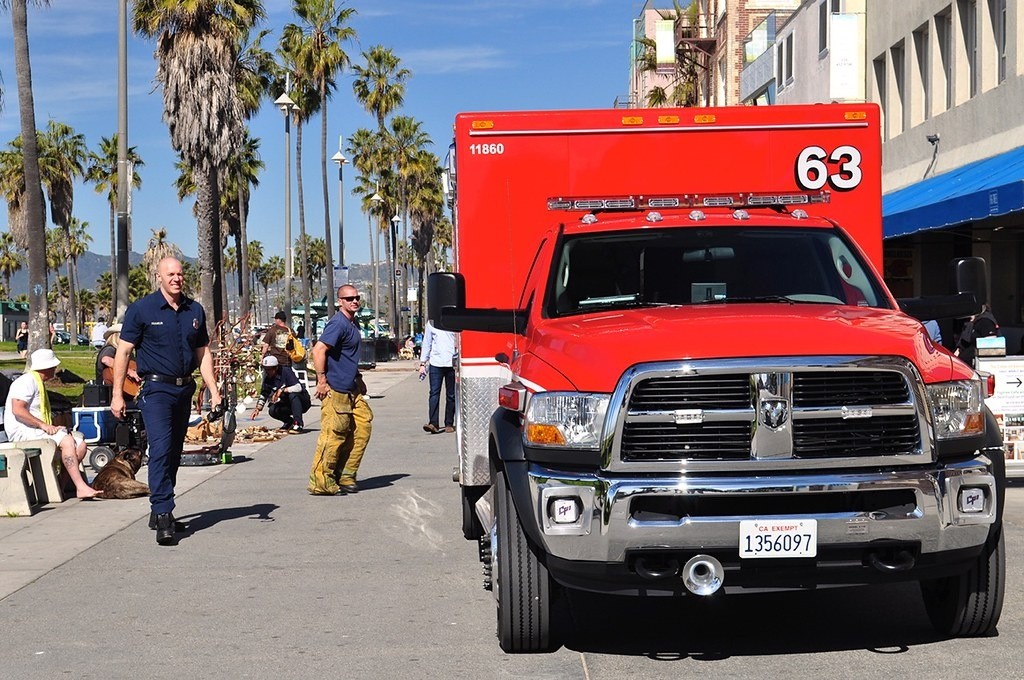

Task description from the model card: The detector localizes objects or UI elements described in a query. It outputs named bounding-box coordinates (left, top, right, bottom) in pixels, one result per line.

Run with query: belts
left=145, top=372, right=192, bottom=386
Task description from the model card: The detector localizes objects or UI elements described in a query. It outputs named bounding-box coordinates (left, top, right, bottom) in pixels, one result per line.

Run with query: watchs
left=316, top=371, right=326, bottom=377
left=419, top=363, right=427, bottom=368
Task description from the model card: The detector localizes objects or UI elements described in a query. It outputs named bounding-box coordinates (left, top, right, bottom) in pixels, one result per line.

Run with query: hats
left=30, top=349, right=61, bottom=371
left=275, top=311, right=286, bottom=320
left=103, top=324, right=123, bottom=339
left=97, top=317, right=104, bottom=322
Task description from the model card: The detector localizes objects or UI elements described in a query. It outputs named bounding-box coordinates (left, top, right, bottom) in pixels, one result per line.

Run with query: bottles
left=419, top=365, right=430, bottom=382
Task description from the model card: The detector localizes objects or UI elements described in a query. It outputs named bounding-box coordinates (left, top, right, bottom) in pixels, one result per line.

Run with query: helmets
left=262, top=356, right=279, bottom=367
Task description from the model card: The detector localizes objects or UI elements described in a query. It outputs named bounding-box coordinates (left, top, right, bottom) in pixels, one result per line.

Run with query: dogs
left=90, top=447, right=150, bottom=499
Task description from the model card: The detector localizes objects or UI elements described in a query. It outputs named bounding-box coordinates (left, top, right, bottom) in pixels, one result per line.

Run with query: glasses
left=341, top=296, right=360, bottom=301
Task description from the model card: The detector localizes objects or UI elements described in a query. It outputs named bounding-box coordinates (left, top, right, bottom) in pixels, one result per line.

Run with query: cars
left=55, top=332, right=89, bottom=346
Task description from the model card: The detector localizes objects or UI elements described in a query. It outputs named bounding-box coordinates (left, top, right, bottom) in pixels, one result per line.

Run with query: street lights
left=276, top=92, right=303, bottom=327
left=332, top=151, right=350, bottom=266
left=370, top=193, right=385, bottom=338
left=391, top=215, right=403, bottom=337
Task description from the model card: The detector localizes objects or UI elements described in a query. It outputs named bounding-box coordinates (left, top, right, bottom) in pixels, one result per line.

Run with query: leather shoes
left=149, top=512, right=186, bottom=533
left=156, top=513, right=175, bottom=543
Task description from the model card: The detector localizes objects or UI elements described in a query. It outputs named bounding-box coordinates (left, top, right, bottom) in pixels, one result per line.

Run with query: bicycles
left=91, top=345, right=101, bottom=359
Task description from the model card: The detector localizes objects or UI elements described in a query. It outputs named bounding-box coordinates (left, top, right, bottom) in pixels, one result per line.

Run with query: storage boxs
left=71, top=385, right=116, bottom=446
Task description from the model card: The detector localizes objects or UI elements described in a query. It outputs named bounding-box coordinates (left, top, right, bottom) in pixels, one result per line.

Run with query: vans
left=311, top=321, right=389, bottom=338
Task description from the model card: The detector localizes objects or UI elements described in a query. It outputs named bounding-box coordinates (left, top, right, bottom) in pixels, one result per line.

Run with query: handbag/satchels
left=285, top=326, right=306, bottom=363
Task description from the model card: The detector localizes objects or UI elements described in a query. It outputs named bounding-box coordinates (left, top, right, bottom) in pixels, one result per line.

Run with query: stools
left=295, top=370, right=311, bottom=395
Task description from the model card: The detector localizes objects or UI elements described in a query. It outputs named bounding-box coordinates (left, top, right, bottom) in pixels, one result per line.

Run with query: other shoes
left=445, top=426, right=454, bottom=432
left=310, top=486, right=348, bottom=497
left=423, top=424, right=439, bottom=433
left=279, top=418, right=293, bottom=431
left=289, top=418, right=304, bottom=434
left=339, top=478, right=359, bottom=493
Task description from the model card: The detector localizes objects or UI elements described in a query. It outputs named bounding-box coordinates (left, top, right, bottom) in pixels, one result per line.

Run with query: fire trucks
left=428, top=105, right=1005, bottom=652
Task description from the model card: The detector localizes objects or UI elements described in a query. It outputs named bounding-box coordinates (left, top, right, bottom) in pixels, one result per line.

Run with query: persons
left=417, top=314, right=458, bottom=434
left=90, top=316, right=151, bottom=470
left=250, top=355, right=312, bottom=434
left=259, top=312, right=306, bottom=383
left=918, top=294, right=1003, bottom=369
left=306, top=284, right=373, bottom=496
left=16, top=319, right=56, bottom=359
left=3, top=349, right=104, bottom=499
left=402, top=331, right=425, bottom=360
left=110, top=255, right=222, bottom=543
left=293, top=319, right=306, bottom=339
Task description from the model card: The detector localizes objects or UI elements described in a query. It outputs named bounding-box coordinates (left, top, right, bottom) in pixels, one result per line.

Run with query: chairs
left=0, top=406, right=89, bottom=516
